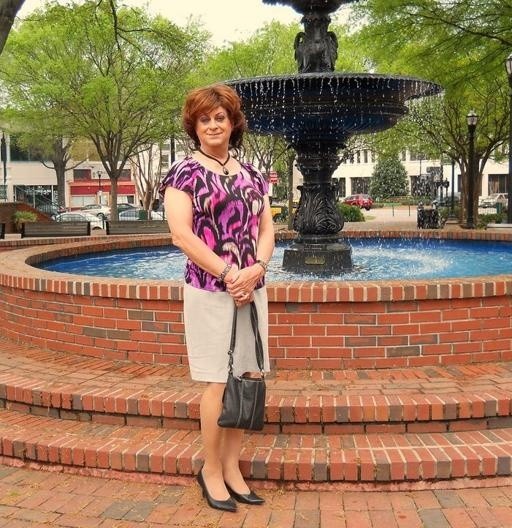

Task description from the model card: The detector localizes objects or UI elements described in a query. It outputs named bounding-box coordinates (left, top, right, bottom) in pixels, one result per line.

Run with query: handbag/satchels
left=217, top=375, right=265, bottom=431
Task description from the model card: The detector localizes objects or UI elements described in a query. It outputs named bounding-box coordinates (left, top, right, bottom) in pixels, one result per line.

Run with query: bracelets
left=219, top=264, right=232, bottom=281
left=255, top=260, right=268, bottom=272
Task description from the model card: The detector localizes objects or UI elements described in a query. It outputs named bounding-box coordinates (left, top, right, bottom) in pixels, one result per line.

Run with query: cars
left=284, top=199, right=300, bottom=216
left=35, top=202, right=167, bottom=231
left=433, top=196, right=459, bottom=207
left=343, top=194, right=373, bottom=211
left=482, top=192, right=507, bottom=207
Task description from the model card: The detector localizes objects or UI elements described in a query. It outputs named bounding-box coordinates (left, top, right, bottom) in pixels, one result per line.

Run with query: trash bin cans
left=418, top=209, right=438, bottom=229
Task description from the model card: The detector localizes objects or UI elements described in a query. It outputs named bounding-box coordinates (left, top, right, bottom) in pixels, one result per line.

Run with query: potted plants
left=12, top=210, right=38, bottom=233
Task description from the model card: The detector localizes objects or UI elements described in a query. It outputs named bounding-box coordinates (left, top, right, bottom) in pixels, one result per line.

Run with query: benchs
left=106, top=220, right=171, bottom=235
left=21, top=221, right=91, bottom=237
left=0, top=222, right=5, bottom=239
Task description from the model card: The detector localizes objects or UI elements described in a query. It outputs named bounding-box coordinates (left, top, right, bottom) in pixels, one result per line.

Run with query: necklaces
left=198, top=148, right=231, bottom=175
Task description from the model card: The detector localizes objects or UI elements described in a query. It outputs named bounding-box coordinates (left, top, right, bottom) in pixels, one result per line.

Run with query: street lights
left=466, top=110, right=478, bottom=228
left=97, top=170, right=102, bottom=204
left=449, top=154, right=459, bottom=216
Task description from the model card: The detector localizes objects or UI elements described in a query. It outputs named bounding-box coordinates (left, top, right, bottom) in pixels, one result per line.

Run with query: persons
left=162, top=83, right=276, bottom=513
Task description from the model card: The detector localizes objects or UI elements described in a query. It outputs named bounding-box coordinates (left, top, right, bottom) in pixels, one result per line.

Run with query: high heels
left=196, top=465, right=265, bottom=511
